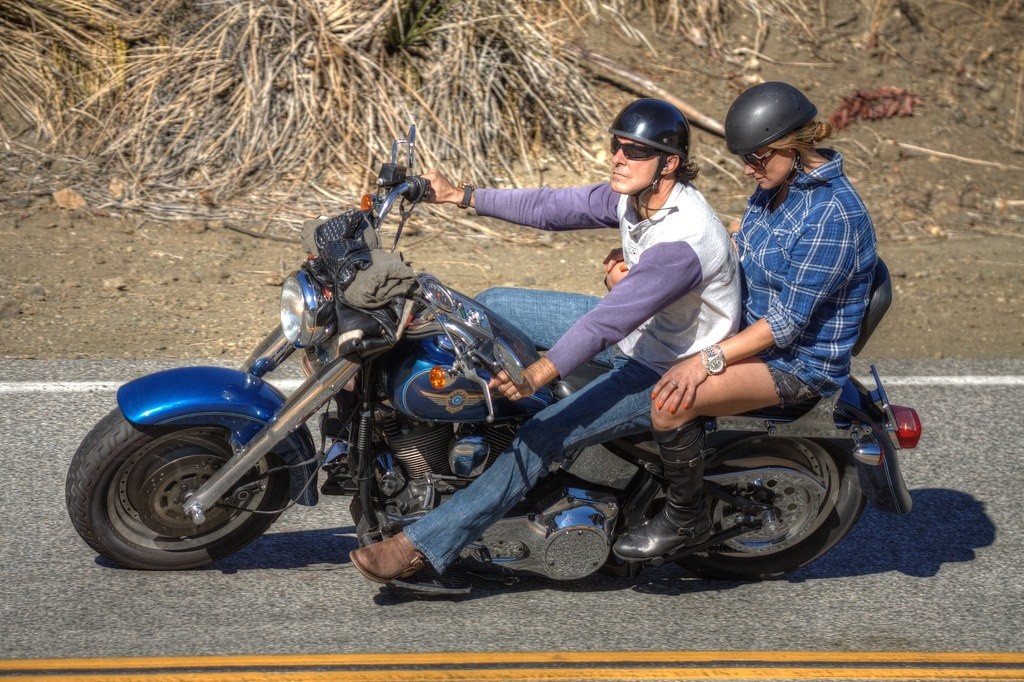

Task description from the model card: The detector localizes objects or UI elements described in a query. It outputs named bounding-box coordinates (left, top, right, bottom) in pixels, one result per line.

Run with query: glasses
left=610, top=138, right=660, bottom=160
left=742, top=149, right=776, bottom=170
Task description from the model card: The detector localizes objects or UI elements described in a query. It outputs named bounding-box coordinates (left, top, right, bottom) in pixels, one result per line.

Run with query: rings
left=669, top=380, right=678, bottom=389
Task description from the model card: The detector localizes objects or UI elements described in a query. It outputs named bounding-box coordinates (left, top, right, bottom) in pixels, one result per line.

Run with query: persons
left=348, top=96, right=745, bottom=587
left=602, top=81, right=878, bottom=565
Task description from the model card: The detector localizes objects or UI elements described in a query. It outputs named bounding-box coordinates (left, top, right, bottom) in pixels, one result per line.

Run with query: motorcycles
left=66, top=123, right=922, bottom=597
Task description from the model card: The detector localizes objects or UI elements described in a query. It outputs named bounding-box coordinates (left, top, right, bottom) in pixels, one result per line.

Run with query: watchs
left=703, top=346, right=724, bottom=376
left=456, top=180, right=476, bottom=209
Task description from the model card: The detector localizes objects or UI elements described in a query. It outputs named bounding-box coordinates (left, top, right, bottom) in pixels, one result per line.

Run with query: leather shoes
left=348, top=531, right=426, bottom=584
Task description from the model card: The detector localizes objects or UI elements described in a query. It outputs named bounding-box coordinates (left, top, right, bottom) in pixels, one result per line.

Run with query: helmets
left=725, top=81, right=817, bottom=156
left=608, top=98, right=691, bottom=161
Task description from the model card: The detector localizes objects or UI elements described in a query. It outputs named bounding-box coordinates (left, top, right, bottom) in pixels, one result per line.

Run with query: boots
left=613, top=414, right=713, bottom=562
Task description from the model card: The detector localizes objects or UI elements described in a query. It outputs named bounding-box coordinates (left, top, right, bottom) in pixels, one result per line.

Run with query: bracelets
left=714, top=343, right=729, bottom=366
left=699, top=349, right=714, bottom=376
